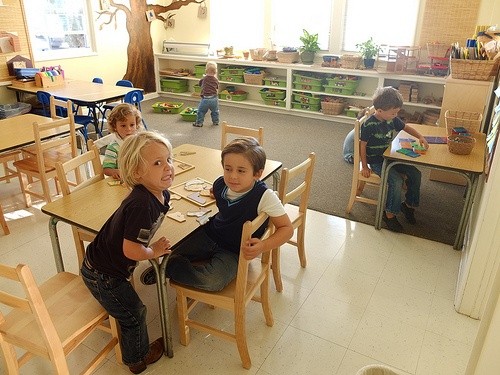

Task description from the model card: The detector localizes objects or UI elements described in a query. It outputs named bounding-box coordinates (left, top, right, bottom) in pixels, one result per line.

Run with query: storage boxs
left=151, top=64, right=363, bottom=123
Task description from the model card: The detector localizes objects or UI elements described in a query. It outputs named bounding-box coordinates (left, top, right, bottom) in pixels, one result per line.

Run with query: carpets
left=72, top=96, right=467, bottom=247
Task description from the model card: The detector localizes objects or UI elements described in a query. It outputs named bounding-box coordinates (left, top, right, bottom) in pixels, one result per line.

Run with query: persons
left=139, top=135, right=294, bottom=291
left=102, top=104, right=141, bottom=180
left=193, top=60, right=220, bottom=127
left=81, top=131, right=174, bottom=375
left=360, top=86, right=430, bottom=232
left=344, top=107, right=369, bottom=164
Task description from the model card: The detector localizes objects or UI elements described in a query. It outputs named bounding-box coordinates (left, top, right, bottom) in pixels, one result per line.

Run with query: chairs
left=0, top=77, right=390, bottom=375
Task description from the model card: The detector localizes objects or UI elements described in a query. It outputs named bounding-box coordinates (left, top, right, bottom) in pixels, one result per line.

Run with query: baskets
left=444, top=110, right=482, bottom=135
left=450, top=58, right=495, bottom=81
left=277, top=51, right=300, bottom=63
left=320, top=102, right=344, bottom=115
left=243, top=71, right=269, bottom=84
left=447, top=135, right=476, bottom=155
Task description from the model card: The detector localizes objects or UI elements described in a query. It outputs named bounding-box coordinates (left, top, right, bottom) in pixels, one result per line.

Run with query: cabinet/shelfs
left=153, top=54, right=446, bottom=126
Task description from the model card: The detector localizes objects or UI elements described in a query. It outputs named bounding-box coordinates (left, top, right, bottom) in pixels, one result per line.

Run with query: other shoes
left=213, top=123, right=219, bottom=126
left=193, top=122, right=203, bottom=127
left=400, top=201, right=416, bottom=224
left=382, top=210, right=403, bottom=232
left=140, top=264, right=160, bottom=285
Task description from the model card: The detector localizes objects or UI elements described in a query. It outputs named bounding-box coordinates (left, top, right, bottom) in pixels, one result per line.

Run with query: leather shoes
left=129, top=337, right=164, bottom=374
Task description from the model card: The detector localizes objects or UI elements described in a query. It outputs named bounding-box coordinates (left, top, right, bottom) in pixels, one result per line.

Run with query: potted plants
left=297, top=28, right=321, bottom=65
left=356, top=37, right=382, bottom=69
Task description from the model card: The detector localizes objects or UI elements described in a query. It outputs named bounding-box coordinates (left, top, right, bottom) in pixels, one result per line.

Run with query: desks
left=374, top=123, right=487, bottom=251
left=0, top=113, right=84, bottom=195
left=8, top=81, right=143, bottom=140
left=41, top=143, right=283, bottom=299
